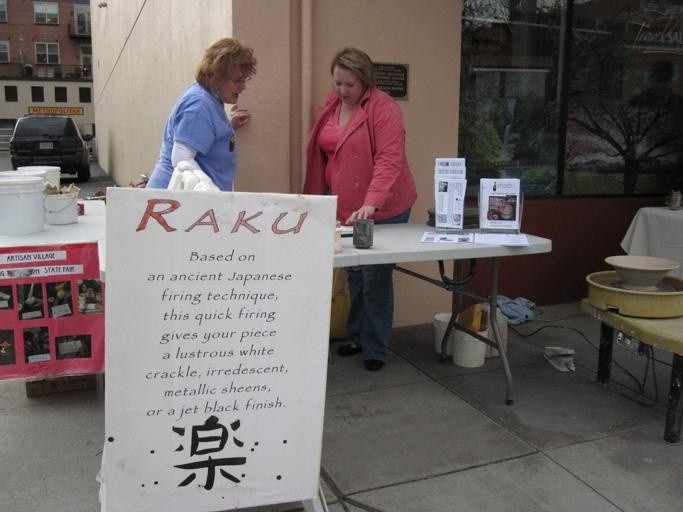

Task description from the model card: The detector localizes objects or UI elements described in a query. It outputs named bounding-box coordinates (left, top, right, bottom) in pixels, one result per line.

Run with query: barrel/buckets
left=17, top=166, right=62, bottom=190
left=0, top=169, right=47, bottom=182
left=433, top=311, right=452, bottom=356
left=0, top=175, right=46, bottom=237
left=43, top=186, right=80, bottom=225
left=452, top=327, right=489, bottom=369
left=481, top=301, right=507, bottom=356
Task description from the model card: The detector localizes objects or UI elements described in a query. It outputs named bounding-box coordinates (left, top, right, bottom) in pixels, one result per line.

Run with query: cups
left=352, top=219, right=374, bottom=249
left=665, top=191, right=683, bottom=210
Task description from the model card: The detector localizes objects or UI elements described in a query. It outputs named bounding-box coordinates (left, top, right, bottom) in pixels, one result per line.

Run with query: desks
left=0, top=198, right=107, bottom=274
left=635, top=205, right=683, bottom=280
left=328, top=221, right=552, bottom=405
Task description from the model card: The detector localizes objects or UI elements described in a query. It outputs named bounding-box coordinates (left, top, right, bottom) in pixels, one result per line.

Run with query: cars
left=9, top=115, right=92, bottom=182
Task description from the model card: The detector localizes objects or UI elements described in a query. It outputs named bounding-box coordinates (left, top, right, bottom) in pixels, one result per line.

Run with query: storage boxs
left=24, top=373, right=97, bottom=397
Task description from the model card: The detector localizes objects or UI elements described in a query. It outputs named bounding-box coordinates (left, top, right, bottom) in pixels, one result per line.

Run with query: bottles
left=77, top=199, right=84, bottom=215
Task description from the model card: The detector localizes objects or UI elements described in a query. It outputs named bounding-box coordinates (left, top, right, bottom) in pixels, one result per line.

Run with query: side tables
left=578, top=296, right=683, bottom=445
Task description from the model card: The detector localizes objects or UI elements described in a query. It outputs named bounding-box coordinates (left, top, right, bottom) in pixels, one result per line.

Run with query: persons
left=144, top=38, right=258, bottom=191
left=302, top=48, right=418, bottom=371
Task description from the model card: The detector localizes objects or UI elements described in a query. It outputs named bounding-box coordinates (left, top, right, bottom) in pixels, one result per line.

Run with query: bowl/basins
left=604, top=254, right=680, bottom=291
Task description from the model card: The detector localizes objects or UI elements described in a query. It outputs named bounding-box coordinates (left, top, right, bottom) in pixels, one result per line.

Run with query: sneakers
left=338, top=341, right=361, bottom=357
left=365, top=359, right=383, bottom=371
